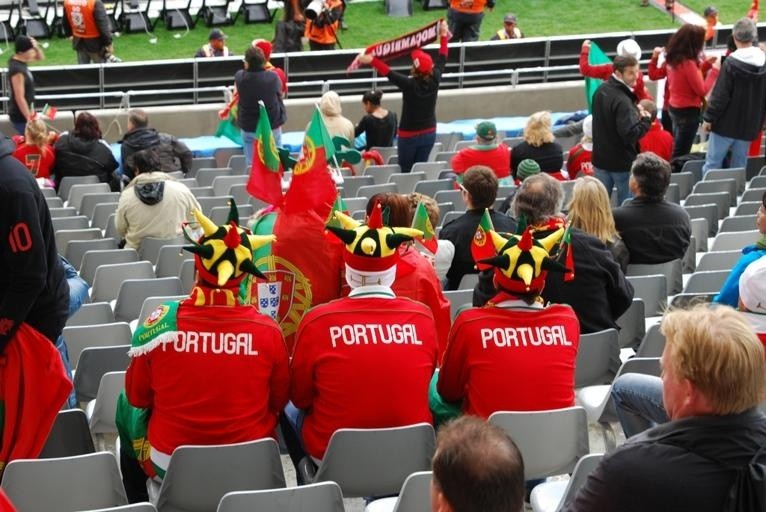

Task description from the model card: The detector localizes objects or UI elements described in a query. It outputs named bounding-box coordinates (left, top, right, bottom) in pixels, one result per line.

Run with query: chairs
left=484, top=405, right=593, bottom=488
left=214, top=478, right=350, bottom=511
left=297, top=422, right=439, bottom=497
left=0, top=123, right=765, bottom=451
left=529, top=450, right=606, bottom=511
left=144, top=436, right=290, bottom=509
left=361, top=469, right=434, bottom=511
left=1, top=451, right=130, bottom=511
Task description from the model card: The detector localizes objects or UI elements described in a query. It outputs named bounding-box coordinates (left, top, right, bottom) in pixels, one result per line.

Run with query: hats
left=323, top=200, right=425, bottom=289
left=246, top=38, right=273, bottom=60
left=475, top=120, right=498, bottom=142
left=177, top=195, right=270, bottom=291
left=474, top=223, right=566, bottom=305
left=320, top=91, right=341, bottom=113
left=14, top=34, right=33, bottom=54
left=516, top=158, right=541, bottom=180
left=503, top=14, right=517, bottom=24
left=411, top=48, right=435, bottom=76
left=208, top=29, right=229, bottom=41
left=616, top=38, right=643, bottom=63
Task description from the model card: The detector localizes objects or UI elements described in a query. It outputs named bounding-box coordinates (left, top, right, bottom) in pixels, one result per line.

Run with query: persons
left=0, top=0, right=766, bottom=512
